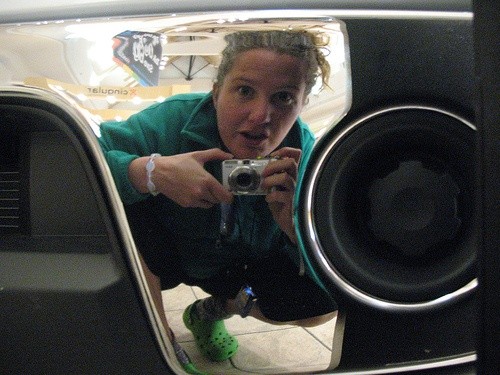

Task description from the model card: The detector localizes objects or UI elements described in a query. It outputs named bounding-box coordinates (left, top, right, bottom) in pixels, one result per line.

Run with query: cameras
left=222, top=159, right=279, bottom=195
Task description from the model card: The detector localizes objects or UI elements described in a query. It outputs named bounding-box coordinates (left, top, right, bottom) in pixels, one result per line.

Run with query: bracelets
left=146, top=152, right=160, bottom=196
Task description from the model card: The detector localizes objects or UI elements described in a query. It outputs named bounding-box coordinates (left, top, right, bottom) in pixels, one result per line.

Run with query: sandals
left=175, top=298, right=239, bottom=375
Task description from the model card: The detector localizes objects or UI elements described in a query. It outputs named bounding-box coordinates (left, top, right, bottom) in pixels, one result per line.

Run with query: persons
left=91, top=26, right=349, bottom=375
